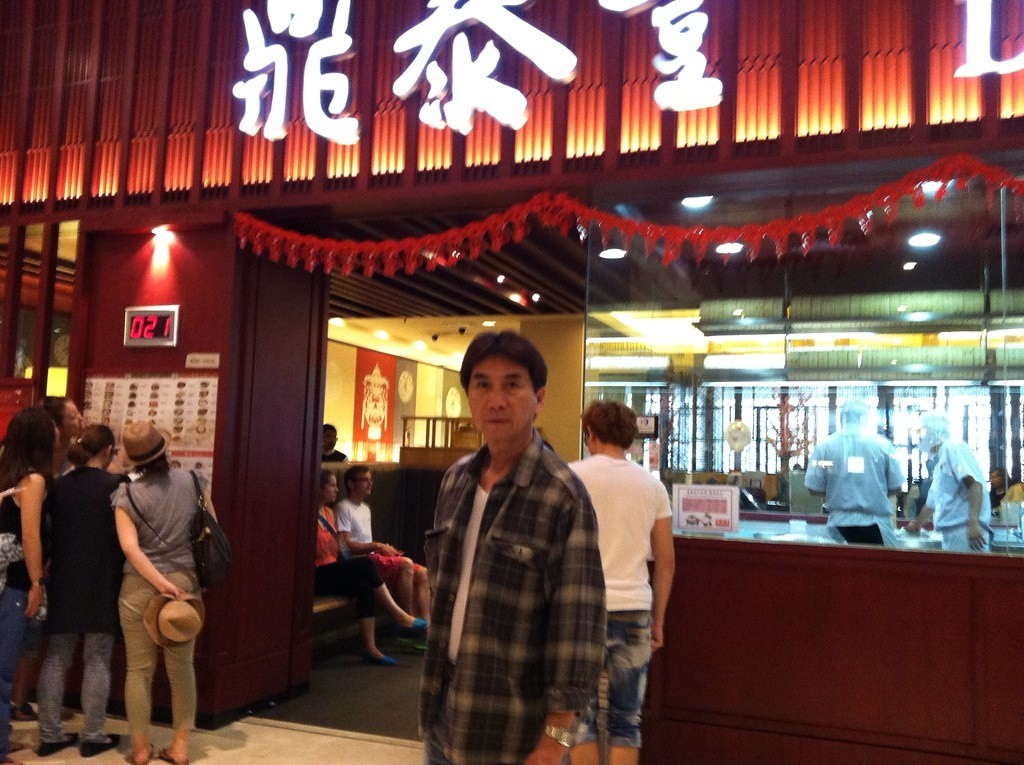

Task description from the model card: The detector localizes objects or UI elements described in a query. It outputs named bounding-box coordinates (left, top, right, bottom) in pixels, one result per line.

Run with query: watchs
left=542, top=721, right=573, bottom=749
left=32, top=577, right=46, bottom=585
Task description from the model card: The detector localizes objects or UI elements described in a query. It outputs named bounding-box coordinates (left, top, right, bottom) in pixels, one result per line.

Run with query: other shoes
left=38, top=732, right=80, bottom=756
left=0, top=741, right=24, bottom=753
left=82, top=733, right=123, bottom=758
left=9, top=702, right=38, bottom=721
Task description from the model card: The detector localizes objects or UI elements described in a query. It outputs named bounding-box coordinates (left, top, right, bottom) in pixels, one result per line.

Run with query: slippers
left=395, top=617, right=427, bottom=629
left=133, top=741, right=156, bottom=765
left=158, top=747, right=189, bottom=765
left=365, top=653, right=398, bottom=666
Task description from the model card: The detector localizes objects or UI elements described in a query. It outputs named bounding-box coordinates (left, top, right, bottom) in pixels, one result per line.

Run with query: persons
left=417, top=330, right=608, bottom=765
left=314, top=424, right=433, bottom=665
left=563, top=398, right=677, bottom=765
left=988, top=468, right=1024, bottom=515
left=804, top=397, right=907, bottom=548
left=0, top=396, right=219, bottom=765
left=902, top=414, right=995, bottom=553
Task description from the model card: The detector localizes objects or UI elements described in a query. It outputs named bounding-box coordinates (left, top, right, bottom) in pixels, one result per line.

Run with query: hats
left=142, top=592, right=205, bottom=648
left=121, top=419, right=172, bottom=466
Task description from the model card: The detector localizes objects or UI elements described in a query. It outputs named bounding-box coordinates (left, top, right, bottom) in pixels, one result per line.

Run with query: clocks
left=54, top=333, right=70, bottom=366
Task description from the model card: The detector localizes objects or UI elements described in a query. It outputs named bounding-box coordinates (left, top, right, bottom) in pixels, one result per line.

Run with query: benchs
left=314, top=584, right=395, bottom=616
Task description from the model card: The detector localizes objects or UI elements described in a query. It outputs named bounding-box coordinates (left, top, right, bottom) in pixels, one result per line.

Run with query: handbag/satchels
left=187, top=471, right=232, bottom=587
left=336, top=548, right=350, bottom=561
